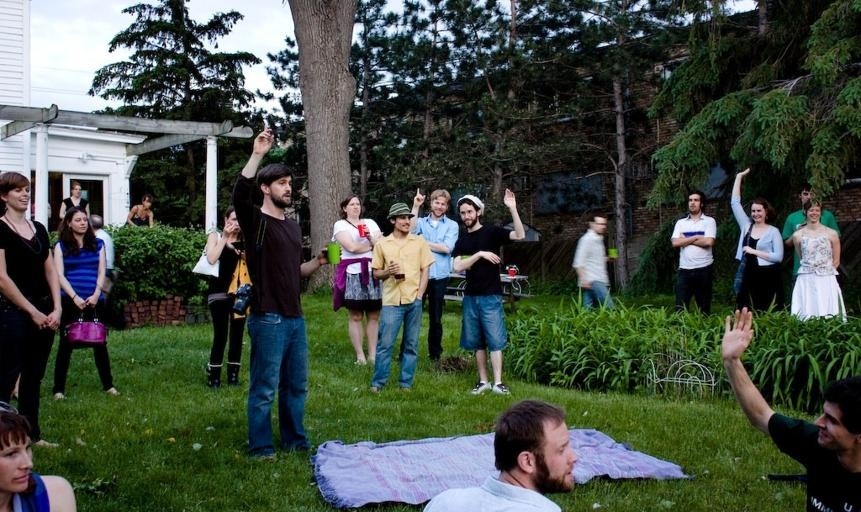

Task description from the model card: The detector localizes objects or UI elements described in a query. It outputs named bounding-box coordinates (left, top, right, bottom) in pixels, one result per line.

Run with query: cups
left=460, top=255, right=471, bottom=270
left=608, top=248, right=617, bottom=258
left=327, top=242, right=341, bottom=265
left=391, top=261, right=405, bottom=284
left=356, top=224, right=367, bottom=238
left=508, top=269, right=515, bottom=277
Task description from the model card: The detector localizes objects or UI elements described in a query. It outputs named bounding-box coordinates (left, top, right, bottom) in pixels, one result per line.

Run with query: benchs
left=446, top=271, right=537, bottom=314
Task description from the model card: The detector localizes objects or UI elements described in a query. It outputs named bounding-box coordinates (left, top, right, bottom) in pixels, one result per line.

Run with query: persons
left=572, top=215, right=615, bottom=310
left=722, top=307, right=861, bottom=512
left=452, top=189, right=526, bottom=396
left=1, top=410, right=77, bottom=512
left=731, top=168, right=848, bottom=324
left=332, top=188, right=459, bottom=394
left=424, top=400, right=578, bottom=512
left=126, top=194, right=154, bottom=228
left=671, top=194, right=717, bottom=315
left=0, top=173, right=119, bottom=446
left=208, top=129, right=342, bottom=457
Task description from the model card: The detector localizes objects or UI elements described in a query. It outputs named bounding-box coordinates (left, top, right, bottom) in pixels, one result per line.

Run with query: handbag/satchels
left=64, top=306, right=109, bottom=346
left=191, top=232, right=224, bottom=279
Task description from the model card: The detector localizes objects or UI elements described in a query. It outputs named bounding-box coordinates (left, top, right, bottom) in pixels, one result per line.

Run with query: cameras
left=231, top=284, right=252, bottom=315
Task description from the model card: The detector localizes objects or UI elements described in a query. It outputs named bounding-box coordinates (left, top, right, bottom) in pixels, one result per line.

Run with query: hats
left=457, top=194, right=486, bottom=217
left=386, top=202, right=415, bottom=220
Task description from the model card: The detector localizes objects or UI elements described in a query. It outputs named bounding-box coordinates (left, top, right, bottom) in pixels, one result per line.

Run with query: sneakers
left=53, top=392, right=68, bottom=402
left=471, top=381, right=492, bottom=396
left=35, top=440, right=60, bottom=449
left=353, top=355, right=441, bottom=397
left=492, top=382, right=511, bottom=395
left=107, top=387, right=121, bottom=396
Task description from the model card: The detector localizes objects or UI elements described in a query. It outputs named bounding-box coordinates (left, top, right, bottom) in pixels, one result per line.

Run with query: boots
left=228, top=362, right=241, bottom=389
left=207, top=364, right=221, bottom=391
